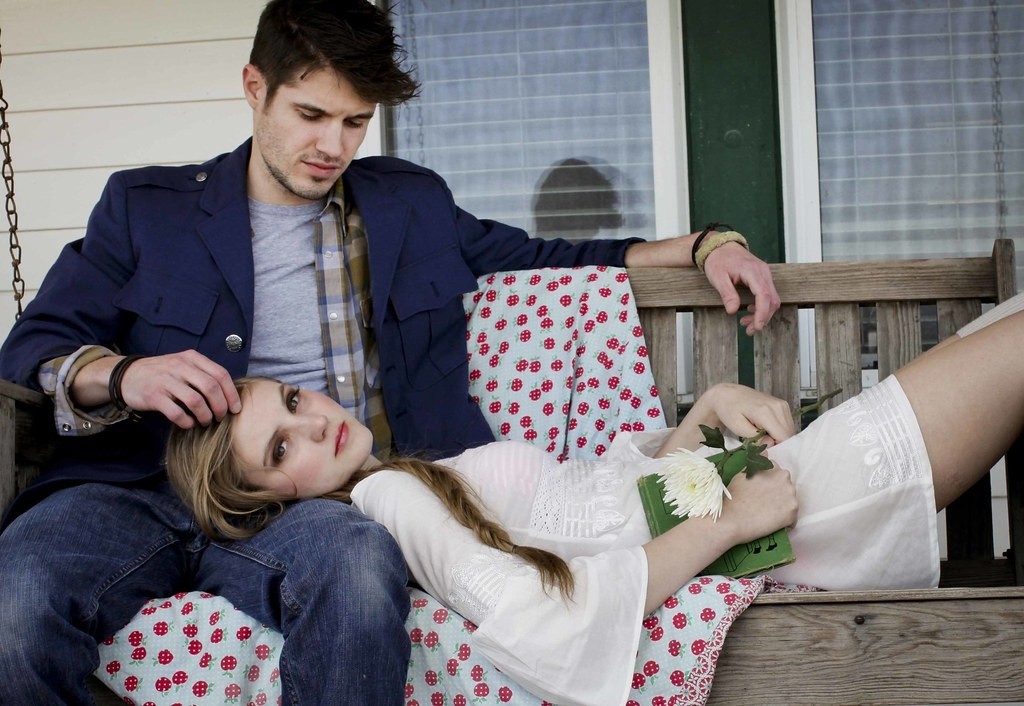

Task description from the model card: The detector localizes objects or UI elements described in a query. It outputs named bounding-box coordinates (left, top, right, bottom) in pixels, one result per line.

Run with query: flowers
left=655, top=388, right=845, bottom=524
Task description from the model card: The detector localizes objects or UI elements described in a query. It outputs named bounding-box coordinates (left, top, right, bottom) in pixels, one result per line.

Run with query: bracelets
left=692, top=223, right=749, bottom=273
left=109, top=352, right=156, bottom=421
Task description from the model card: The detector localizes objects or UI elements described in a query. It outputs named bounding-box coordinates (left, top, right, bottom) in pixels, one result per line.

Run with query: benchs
left=0, top=240, right=1024, bottom=706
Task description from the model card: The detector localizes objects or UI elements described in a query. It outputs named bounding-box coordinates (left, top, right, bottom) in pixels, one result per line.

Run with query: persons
left=167, top=290, right=1023, bottom=706
left=1, top=0, right=780, bottom=706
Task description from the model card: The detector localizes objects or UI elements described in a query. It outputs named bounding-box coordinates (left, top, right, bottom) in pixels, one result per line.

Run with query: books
left=638, top=445, right=796, bottom=580
